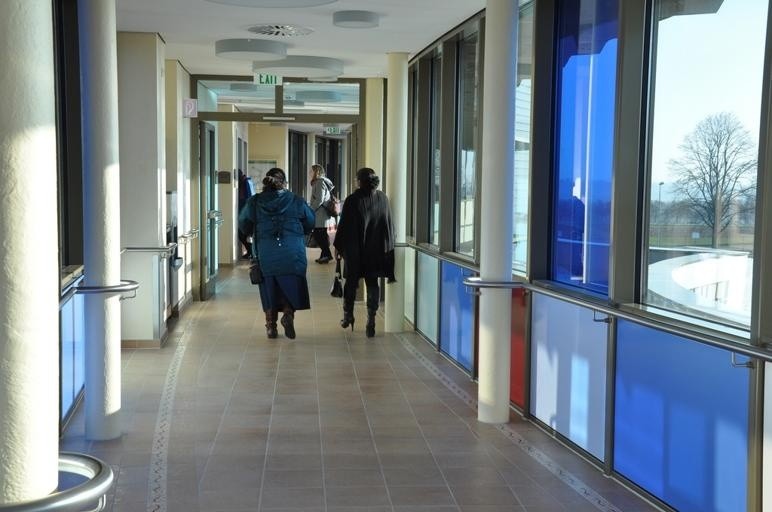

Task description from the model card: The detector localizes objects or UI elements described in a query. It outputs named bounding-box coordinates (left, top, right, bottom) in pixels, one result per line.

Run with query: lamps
left=283, top=96, right=304, bottom=107
left=333, top=10, right=380, bottom=28
left=295, top=91, right=341, bottom=102
left=252, top=54, right=344, bottom=79
left=231, top=83, right=258, bottom=92
left=215, top=39, right=286, bottom=62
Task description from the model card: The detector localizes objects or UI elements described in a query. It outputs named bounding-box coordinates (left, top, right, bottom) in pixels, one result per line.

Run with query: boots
left=281, top=311, right=295, bottom=340
left=265, top=322, right=278, bottom=338
left=340, top=297, right=354, bottom=331
left=365, top=308, right=375, bottom=338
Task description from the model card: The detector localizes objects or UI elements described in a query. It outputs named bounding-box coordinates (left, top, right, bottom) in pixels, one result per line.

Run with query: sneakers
left=315, top=253, right=333, bottom=264
left=240, top=251, right=253, bottom=261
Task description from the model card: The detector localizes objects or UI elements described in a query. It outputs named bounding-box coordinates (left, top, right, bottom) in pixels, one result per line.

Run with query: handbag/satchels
left=304, top=232, right=331, bottom=248
left=249, top=265, right=263, bottom=284
left=331, top=276, right=342, bottom=298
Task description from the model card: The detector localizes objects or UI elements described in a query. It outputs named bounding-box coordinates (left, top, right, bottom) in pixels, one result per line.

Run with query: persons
left=306, top=164, right=335, bottom=267
left=237, top=167, right=317, bottom=341
left=237, top=167, right=253, bottom=260
left=332, top=167, right=398, bottom=339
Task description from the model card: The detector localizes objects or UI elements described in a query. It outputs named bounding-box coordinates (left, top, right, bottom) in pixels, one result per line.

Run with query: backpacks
left=312, top=180, right=340, bottom=218
